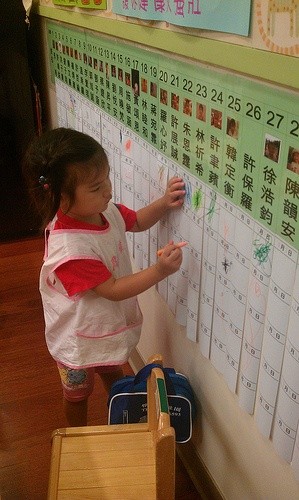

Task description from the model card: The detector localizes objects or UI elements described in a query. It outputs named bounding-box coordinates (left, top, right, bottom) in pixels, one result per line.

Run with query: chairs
left=48, top=354, right=176, bottom=500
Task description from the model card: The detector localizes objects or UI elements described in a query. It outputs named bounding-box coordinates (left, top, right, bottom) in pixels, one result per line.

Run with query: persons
left=23, top=126, right=186, bottom=426
left=50, top=40, right=299, bottom=176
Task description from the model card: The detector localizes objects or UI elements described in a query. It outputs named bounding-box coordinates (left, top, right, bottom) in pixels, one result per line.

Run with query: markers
left=156, top=241, right=189, bottom=257
